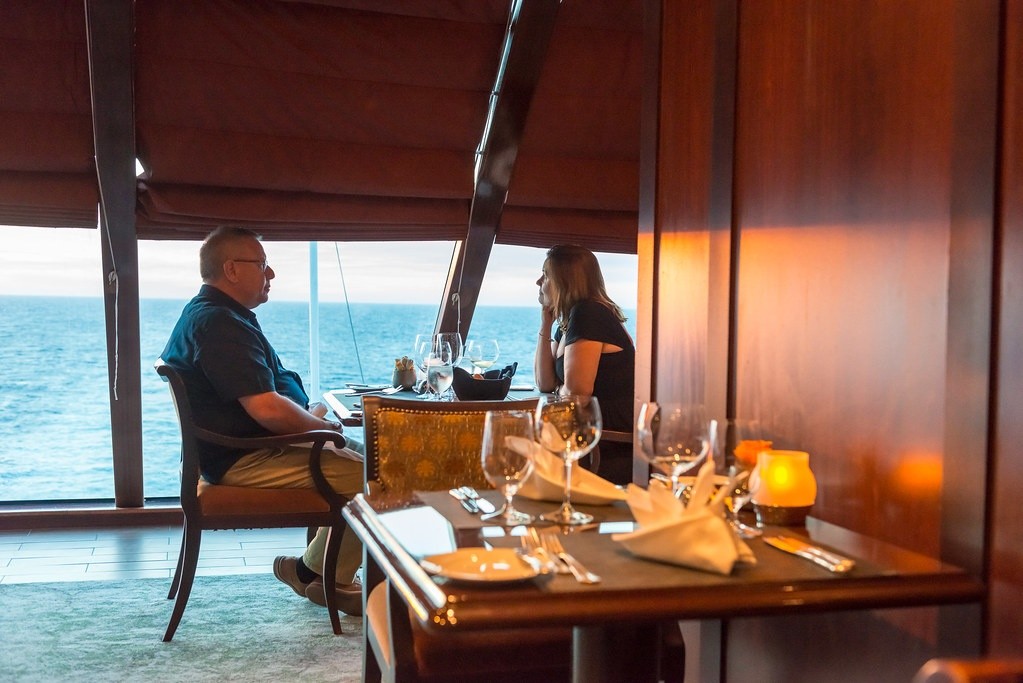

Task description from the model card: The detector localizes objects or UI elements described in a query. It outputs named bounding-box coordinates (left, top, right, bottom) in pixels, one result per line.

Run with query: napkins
left=361, top=395, right=571, bottom=683
left=611, top=474, right=759, bottom=575
left=504, top=436, right=627, bottom=505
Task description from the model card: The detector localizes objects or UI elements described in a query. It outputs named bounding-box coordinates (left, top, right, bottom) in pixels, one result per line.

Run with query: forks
left=344, top=384, right=403, bottom=397
left=539, top=533, right=601, bottom=585
left=520, top=528, right=566, bottom=574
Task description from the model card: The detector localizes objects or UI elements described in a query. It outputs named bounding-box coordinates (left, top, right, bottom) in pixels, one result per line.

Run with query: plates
left=420, top=546, right=540, bottom=583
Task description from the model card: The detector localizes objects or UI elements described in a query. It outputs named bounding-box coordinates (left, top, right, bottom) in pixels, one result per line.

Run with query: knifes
left=778, top=535, right=856, bottom=568
left=458, top=487, right=496, bottom=514
left=449, top=488, right=479, bottom=515
left=763, top=538, right=846, bottom=574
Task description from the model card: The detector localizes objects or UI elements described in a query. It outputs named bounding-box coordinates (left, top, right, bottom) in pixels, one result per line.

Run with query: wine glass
left=711, top=419, right=767, bottom=539
left=427, top=351, right=453, bottom=402
left=481, top=410, right=537, bottom=527
left=468, top=339, right=500, bottom=375
left=421, top=341, right=451, bottom=400
left=414, top=334, right=438, bottom=399
left=636, top=399, right=717, bottom=495
left=533, top=396, right=602, bottom=527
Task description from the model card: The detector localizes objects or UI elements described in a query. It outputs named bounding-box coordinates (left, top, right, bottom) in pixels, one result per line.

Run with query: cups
left=435, top=332, right=463, bottom=369
left=392, top=370, right=417, bottom=391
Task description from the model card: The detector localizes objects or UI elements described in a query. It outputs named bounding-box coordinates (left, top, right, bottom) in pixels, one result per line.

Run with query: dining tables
left=322, top=385, right=555, bottom=463
left=342, top=474, right=983, bottom=683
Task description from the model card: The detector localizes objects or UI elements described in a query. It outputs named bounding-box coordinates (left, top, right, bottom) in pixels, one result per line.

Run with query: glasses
left=221, top=257, right=269, bottom=273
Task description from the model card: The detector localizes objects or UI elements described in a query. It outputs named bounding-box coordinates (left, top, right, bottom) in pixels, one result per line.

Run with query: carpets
left=0, top=573, right=363, bottom=683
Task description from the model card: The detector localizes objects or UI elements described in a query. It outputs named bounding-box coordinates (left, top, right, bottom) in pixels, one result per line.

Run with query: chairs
left=591, top=432, right=633, bottom=476
left=154, top=359, right=346, bottom=641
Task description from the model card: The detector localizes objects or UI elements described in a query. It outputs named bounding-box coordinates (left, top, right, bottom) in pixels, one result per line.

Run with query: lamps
left=749, top=451, right=817, bottom=525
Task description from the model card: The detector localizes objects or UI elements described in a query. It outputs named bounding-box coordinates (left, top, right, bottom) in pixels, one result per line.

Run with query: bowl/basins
left=452, top=361, right=518, bottom=401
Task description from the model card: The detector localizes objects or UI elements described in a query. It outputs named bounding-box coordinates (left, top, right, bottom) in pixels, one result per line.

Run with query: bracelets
left=538, top=332, right=556, bottom=342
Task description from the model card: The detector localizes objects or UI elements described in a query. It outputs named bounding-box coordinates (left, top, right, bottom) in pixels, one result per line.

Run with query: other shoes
left=273, top=555, right=307, bottom=597
left=305, top=576, right=363, bottom=617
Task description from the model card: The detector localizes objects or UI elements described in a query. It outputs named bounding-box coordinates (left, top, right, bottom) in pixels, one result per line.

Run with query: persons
left=535, top=245, right=635, bottom=483
left=159, top=226, right=364, bottom=617
